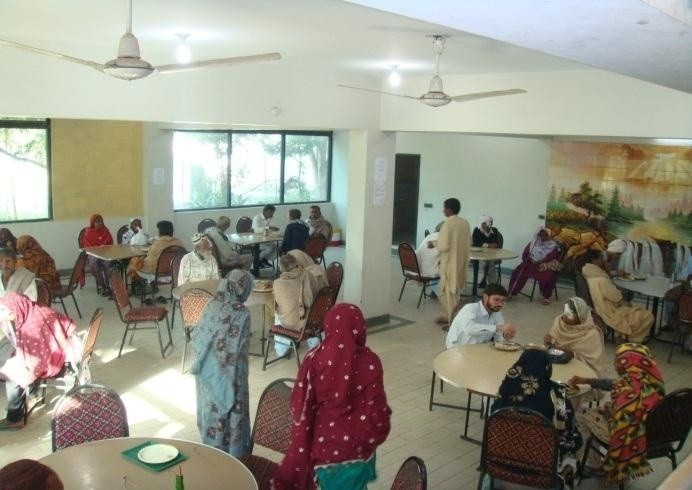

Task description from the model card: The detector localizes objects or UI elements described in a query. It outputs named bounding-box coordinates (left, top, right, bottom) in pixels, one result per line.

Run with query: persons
left=427, top=198, right=470, bottom=331
left=0, top=292, right=76, bottom=432
left=567, top=342, right=665, bottom=486
left=470, top=217, right=503, bottom=289
left=446, top=284, right=515, bottom=348
left=544, top=297, right=607, bottom=402
left=15, top=235, right=63, bottom=291
left=0, top=246, right=38, bottom=309
left=416, top=222, right=442, bottom=295
left=189, top=268, right=250, bottom=460
left=491, top=348, right=583, bottom=472
left=271, top=304, right=392, bottom=490
left=84, top=205, right=333, bottom=357
left=575, top=239, right=654, bottom=342
left=506, top=228, right=563, bottom=306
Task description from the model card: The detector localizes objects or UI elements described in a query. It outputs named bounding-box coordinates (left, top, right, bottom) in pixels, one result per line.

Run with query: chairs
left=666, top=295, right=692, bottom=362
left=397, top=242, right=439, bottom=309
left=519, top=246, right=564, bottom=302
left=574, top=275, right=628, bottom=345
left=52, top=385, right=129, bottom=452
left=240, top=377, right=297, bottom=490
left=576, top=388, right=692, bottom=490
left=390, top=457, right=428, bottom=490
left=478, top=406, right=574, bottom=490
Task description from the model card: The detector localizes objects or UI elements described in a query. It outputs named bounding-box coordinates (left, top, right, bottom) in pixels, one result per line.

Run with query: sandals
left=543, top=297, right=550, bottom=305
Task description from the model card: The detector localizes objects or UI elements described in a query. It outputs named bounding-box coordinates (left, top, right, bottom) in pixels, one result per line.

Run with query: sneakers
left=107, top=289, right=113, bottom=301
left=157, top=296, right=167, bottom=304
left=584, top=464, right=606, bottom=476
left=143, top=298, right=152, bottom=306
left=478, top=280, right=487, bottom=289
left=101, top=285, right=107, bottom=297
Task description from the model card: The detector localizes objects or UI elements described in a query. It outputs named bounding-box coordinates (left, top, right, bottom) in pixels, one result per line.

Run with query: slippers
left=0, top=418, right=23, bottom=432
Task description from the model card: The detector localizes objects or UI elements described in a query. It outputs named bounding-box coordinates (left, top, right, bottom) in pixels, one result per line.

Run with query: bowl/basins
left=253, top=280, right=272, bottom=290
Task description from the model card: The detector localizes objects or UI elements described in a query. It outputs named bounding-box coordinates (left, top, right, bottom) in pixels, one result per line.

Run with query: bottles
left=175, top=475, right=184, bottom=490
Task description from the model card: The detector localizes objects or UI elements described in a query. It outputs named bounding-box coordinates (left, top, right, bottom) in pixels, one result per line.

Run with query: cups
left=263, top=229, right=269, bottom=236
left=670, top=271, right=677, bottom=283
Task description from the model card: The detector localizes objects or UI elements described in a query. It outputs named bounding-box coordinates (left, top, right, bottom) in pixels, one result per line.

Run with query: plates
left=253, top=288, right=272, bottom=292
left=141, top=248, right=148, bottom=252
left=549, top=349, right=566, bottom=354
left=137, top=444, right=179, bottom=465
left=633, top=277, right=647, bottom=280
left=524, top=343, right=550, bottom=351
left=617, top=276, right=634, bottom=281
left=471, top=247, right=482, bottom=252
left=492, top=341, right=520, bottom=351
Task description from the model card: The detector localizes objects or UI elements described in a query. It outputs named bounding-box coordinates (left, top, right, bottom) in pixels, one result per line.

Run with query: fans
left=0, top=1, right=285, bottom=81
left=335, top=35, right=527, bottom=107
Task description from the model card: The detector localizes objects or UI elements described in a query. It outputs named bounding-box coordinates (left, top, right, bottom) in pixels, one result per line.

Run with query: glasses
left=493, top=298, right=506, bottom=304
